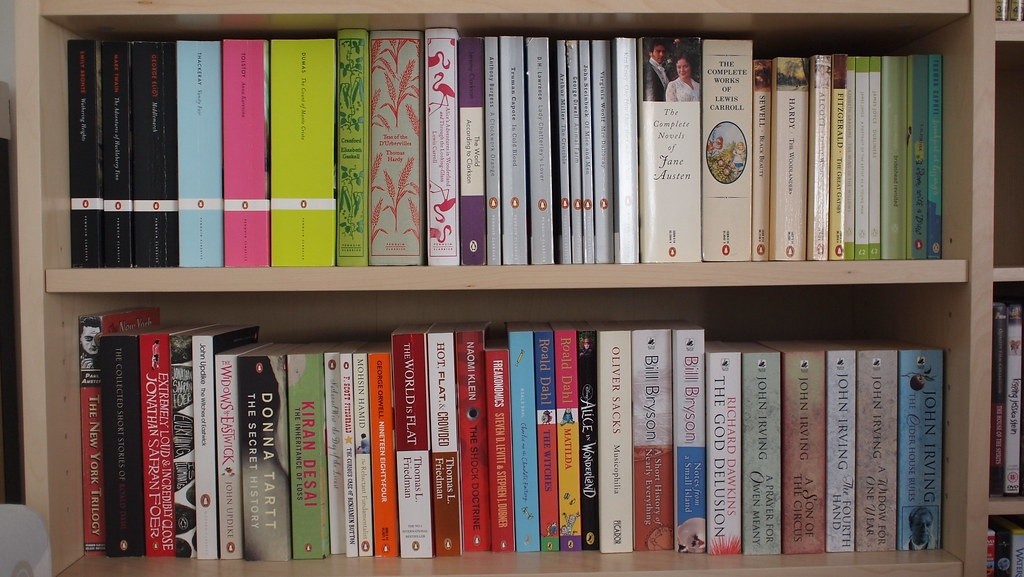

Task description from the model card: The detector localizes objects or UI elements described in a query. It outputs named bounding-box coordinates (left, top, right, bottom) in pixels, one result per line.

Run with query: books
left=78, top=0, right=1024, bottom=577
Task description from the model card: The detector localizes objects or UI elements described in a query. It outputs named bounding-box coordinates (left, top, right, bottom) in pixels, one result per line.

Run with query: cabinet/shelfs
left=15, top=0, right=1024, bottom=577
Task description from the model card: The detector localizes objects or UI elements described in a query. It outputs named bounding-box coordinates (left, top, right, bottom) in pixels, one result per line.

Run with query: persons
left=643, top=40, right=673, bottom=101
left=79, top=315, right=103, bottom=371
left=666, top=52, right=701, bottom=102
left=902, top=506, right=937, bottom=549
